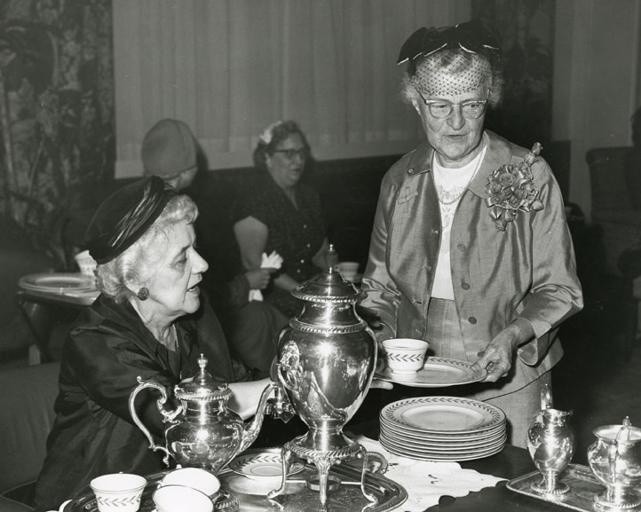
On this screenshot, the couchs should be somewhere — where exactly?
[0,359,67,512]
[44,149,400,272]
[586,147,640,357]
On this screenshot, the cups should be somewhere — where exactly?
[333,261,359,281]
[587,416,640,509]
[382,338,429,374]
[528,407,576,496]
[150,465,222,512]
[90,474,146,512]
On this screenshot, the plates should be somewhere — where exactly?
[378,394,507,463]
[229,477,304,496]
[228,453,304,480]
[375,356,487,388]
[18,273,94,294]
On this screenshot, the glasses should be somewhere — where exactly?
[416,85,488,119]
[275,147,307,159]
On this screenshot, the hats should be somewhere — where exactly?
[84,175,179,264]
[141,118,198,179]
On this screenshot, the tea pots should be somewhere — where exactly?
[131,354,276,469]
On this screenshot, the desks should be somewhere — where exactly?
[15,266,98,341]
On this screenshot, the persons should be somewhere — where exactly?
[75,118,291,384]
[30,174,394,512]
[226,122,365,316]
[356,21,584,453]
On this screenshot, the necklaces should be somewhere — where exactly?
[429,136,485,205]
